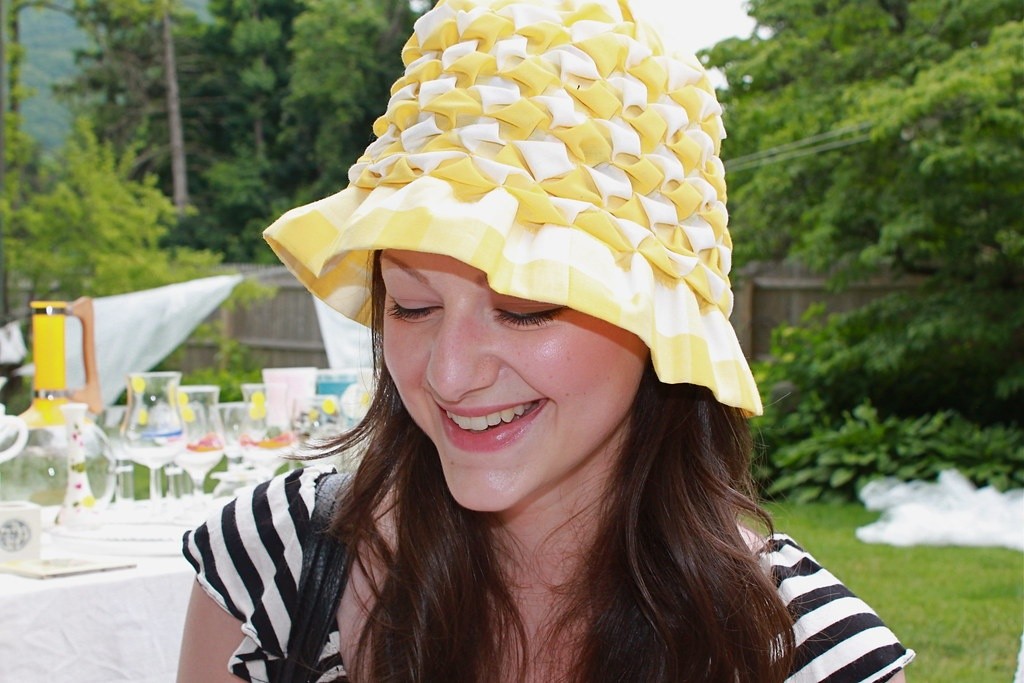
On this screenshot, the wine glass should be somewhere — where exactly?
[105,371,354,522]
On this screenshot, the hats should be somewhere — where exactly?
[263,1,762,419]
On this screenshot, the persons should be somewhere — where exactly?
[177,1,918,683]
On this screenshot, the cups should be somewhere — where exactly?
[262,367,317,427]
[55,403,116,531]
[0,504,41,565]
